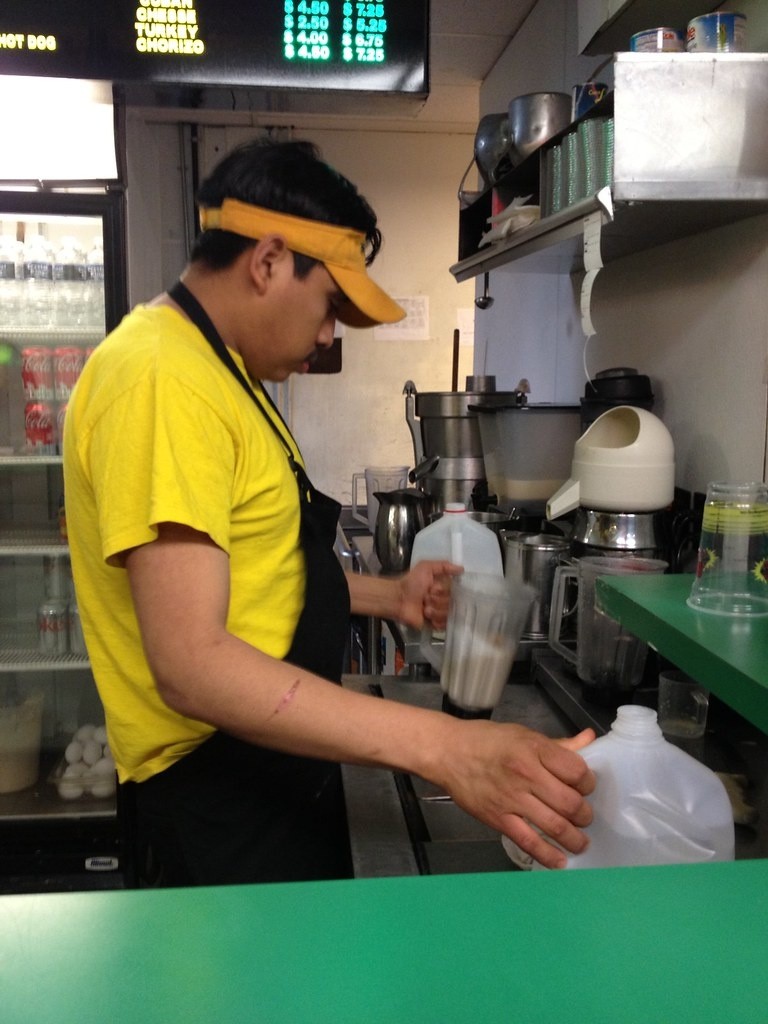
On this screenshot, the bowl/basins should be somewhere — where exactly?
[472,112,511,184]
[508,90,571,157]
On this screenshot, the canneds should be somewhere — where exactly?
[629,27,686,52]
[36,598,87,654]
[686,10,748,52]
[19,344,98,457]
[570,82,608,122]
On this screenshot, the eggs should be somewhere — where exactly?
[59,723,116,799]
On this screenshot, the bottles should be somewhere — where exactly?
[409,503,503,638]
[533,705,736,869]
[0,235,105,327]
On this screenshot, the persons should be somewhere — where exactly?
[62,140,595,889]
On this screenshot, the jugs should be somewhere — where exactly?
[499,526,579,640]
[351,465,410,534]
[372,488,431,575]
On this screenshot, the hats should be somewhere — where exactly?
[198,201,410,328]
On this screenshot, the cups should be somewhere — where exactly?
[418,570,537,713]
[1,686,46,792]
[658,667,710,739]
[685,481,768,618]
[546,555,669,691]
